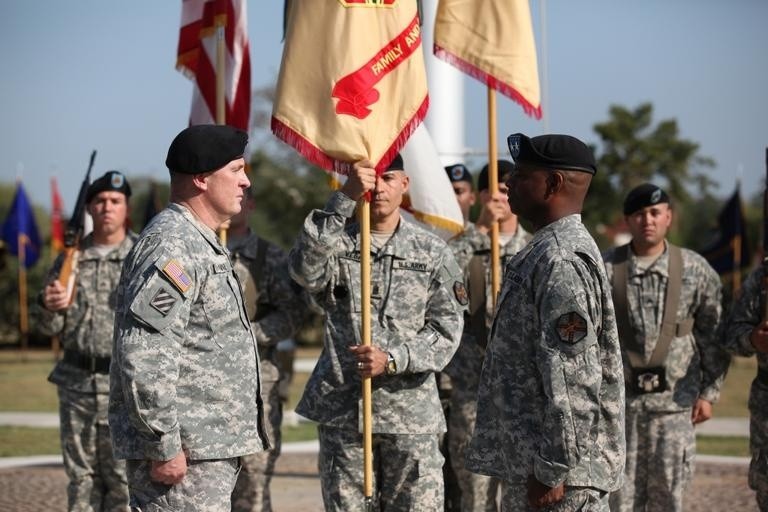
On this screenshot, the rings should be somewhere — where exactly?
[356,362,364,370]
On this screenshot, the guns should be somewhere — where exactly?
[758,145,767,323]
[59,150,97,304]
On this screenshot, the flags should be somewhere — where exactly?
[1,179,42,271]
[269,0,431,203]
[174,1,250,176]
[430,1,542,122]
[41,179,67,262]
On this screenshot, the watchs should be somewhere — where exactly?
[384,354,397,375]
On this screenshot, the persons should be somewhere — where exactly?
[108,124,268,511]
[463,132,625,512]
[227,186,316,510]
[598,184,733,512]
[288,149,470,512]
[435,162,475,230]
[717,250,767,512]
[445,157,527,512]
[28,170,140,512]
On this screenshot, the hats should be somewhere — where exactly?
[166,125,248,174]
[624,184,670,216]
[445,165,472,184]
[478,161,517,192]
[387,154,403,171]
[507,133,597,175]
[86,172,131,204]
